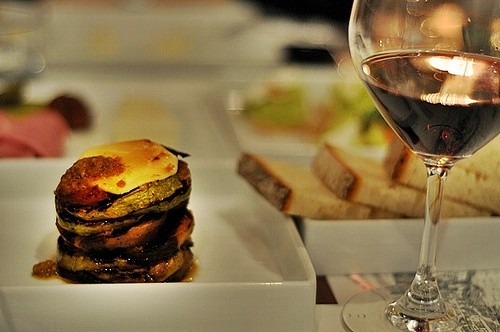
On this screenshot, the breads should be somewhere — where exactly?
[235,128,499,222]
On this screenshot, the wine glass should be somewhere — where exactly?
[339,2,500,331]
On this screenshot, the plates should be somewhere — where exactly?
[279,153,499,272]
[1,155,318,331]
